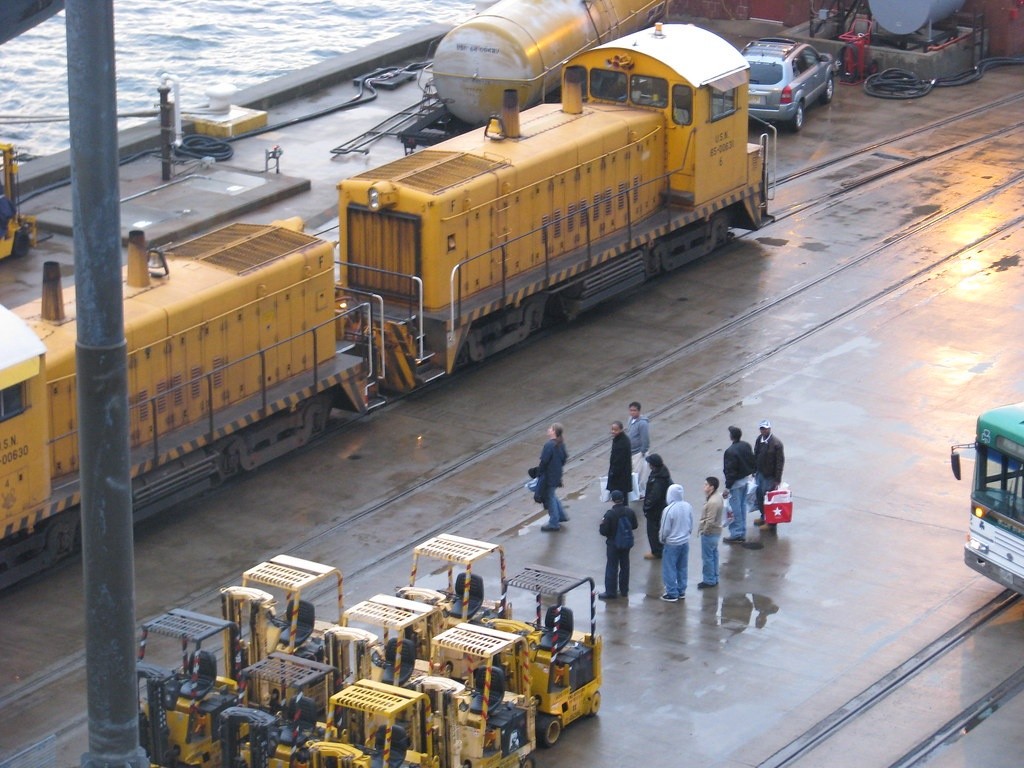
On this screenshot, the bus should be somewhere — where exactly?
[950,402,1024,601]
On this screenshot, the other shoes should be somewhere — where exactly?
[698,582,714,588]
[598,592,616,599]
[559,518,569,522]
[759,524,777,531]
[754,518,765,525]
[664,592,686,598]
[660,595,678,602]
[541,525,560,531]
[644,552,660,559]
[716,582,718,584]
[639,495,644,500]
[621,591,628,597]
[723,536,745,543]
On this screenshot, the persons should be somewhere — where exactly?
[598,490,639,600]
[754,420,785,532]
[659,484,694,602]
[526,422,571,532]
[624,402,652,503]
[696,477,724,589]
[606,420,633,507]
[723,425,757,545]
[642,454,673,561]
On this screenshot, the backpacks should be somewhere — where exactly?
[611,505,634,552]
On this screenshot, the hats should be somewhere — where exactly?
[728,426,742,440]
[608,491,624,500]
[645,454,663,467]
[759,419,771,429]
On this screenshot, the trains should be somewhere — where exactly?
[1,22,777,605]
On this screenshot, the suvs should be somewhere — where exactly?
[741,36,836,132]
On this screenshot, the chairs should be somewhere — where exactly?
[370,725,408,768]
[381,637,416,684]
[449,572,484,619]
[540,606,574,651]
[280,694,317,746]
[280,599,316,647]
[179,650,217,701]
[470,665,506,715]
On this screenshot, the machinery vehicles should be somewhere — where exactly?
[134,535,603,768]
[1,141,38,265]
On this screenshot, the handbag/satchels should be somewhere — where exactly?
[599,472,639,502]
[747,473,760,512]
[721,493,735,528]
[747,476,758,495]
[763,484,793,525]
